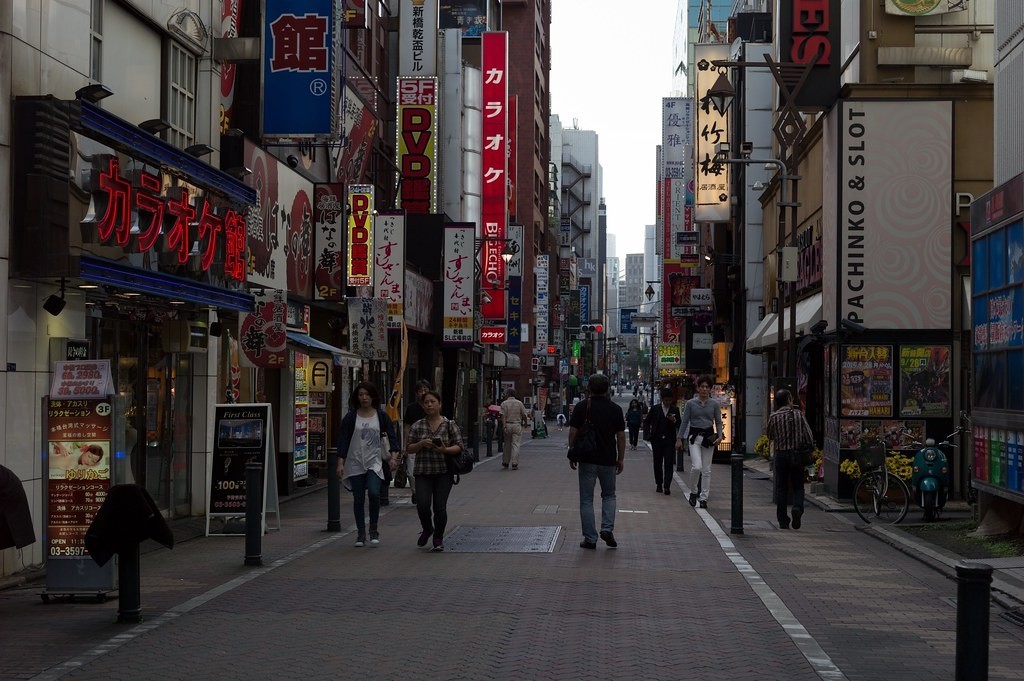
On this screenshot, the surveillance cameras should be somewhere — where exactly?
[483,297,491,304]
[740,142,753,155]
[483,291,493,299]
[714,142,730,154]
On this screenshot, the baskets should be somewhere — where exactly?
[853,444,885,468]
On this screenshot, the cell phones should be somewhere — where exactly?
[433,437,442,449]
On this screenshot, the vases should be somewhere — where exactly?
[858,489,914,505]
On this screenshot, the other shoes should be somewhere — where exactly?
[501,463,509,468]
[512,464,518,470]
[380,497,389,506]
[579,541,596,549]
[630,445,637,451]
[656,485,663,492]
[411,493,417,504]
[780,516,790,529]
[664,487,671,495]
[600,531,617,547]
[369,532,379,544]
[355,537,366,547]
[791,508,802,529]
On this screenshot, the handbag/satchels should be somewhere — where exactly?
[446,419,474,485]
[380,431,391,460]
[798,444,817,466]
[394,455,407,488]
[567,447,582,470]
[707,433,719,446]
[643,426,651,441]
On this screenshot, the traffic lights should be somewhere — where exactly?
[620,351,630,356]
[582,324,603,333]
[531,357,539,372]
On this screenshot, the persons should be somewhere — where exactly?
[638,391,645,402]
[568,373,626,549]
[515,391,524,426]
[643,388,682,495]
[675,374,723,509]
[126,418,137,483]
[407,391,465,551]
[766,388,814,529]
[403,379,431,504]
[625,399,644,450]
[49,443,104,470]
[634,383,638,395]
[336,382,402,546]
[499,388,527,470]
[531,403,539,419]
[557,414,566,432]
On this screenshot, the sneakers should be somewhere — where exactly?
[418,527,434,546]
[433,538,444,551]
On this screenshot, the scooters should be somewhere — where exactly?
[899,425,966,522]
[960,410,987,521]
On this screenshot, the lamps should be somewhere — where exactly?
[210,307,222,338]
[75,84,114,104]
[226,167,252,179]
[44,277,66,316]
[184,144,214,157]
[138,119,170,135]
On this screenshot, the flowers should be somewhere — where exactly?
[840,450,914,489]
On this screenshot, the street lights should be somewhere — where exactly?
[469,238,515,461]
[711,53,819,407]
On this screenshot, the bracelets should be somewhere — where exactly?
[392,457,398,461]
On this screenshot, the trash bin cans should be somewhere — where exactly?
[533,415,546,436]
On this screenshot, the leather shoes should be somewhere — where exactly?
[689,493,697,506]
[700,500,707,508]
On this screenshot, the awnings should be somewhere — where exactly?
[287,332,361,367]
[746,290,823,354]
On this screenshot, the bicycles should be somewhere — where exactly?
[853,432,910,524]
